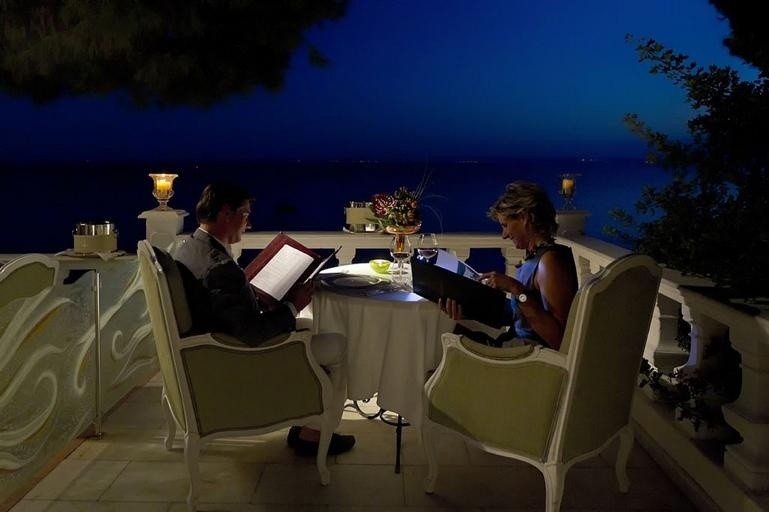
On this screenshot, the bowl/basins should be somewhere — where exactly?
[370,260,391,274]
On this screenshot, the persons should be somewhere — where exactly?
[161,176,358,458]
[436,178,582,357]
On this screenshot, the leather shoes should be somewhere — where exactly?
[287,425,356,457]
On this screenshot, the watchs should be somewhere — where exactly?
[513,291,532,306]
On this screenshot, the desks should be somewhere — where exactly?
[56,256,140,439]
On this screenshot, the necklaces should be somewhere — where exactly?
[513,241,550,269]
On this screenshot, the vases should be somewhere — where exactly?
[385,223,422,264]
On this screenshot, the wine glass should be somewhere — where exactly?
[392,234,413,282]
[416,233,438,263]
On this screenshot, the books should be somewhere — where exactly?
[409,248,508,334]
[243,230,344,310]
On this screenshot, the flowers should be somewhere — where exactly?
[364,170,446,232]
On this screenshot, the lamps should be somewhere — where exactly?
[147,173,179,211]
[558,176,577,212]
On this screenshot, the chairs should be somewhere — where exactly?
[421,253,663,510]
[139,240,335,511]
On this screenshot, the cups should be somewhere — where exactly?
[77,216,115,258]
[349,201,375,233]
[392,259,412,291]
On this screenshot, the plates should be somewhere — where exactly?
[322,273,391,292]
